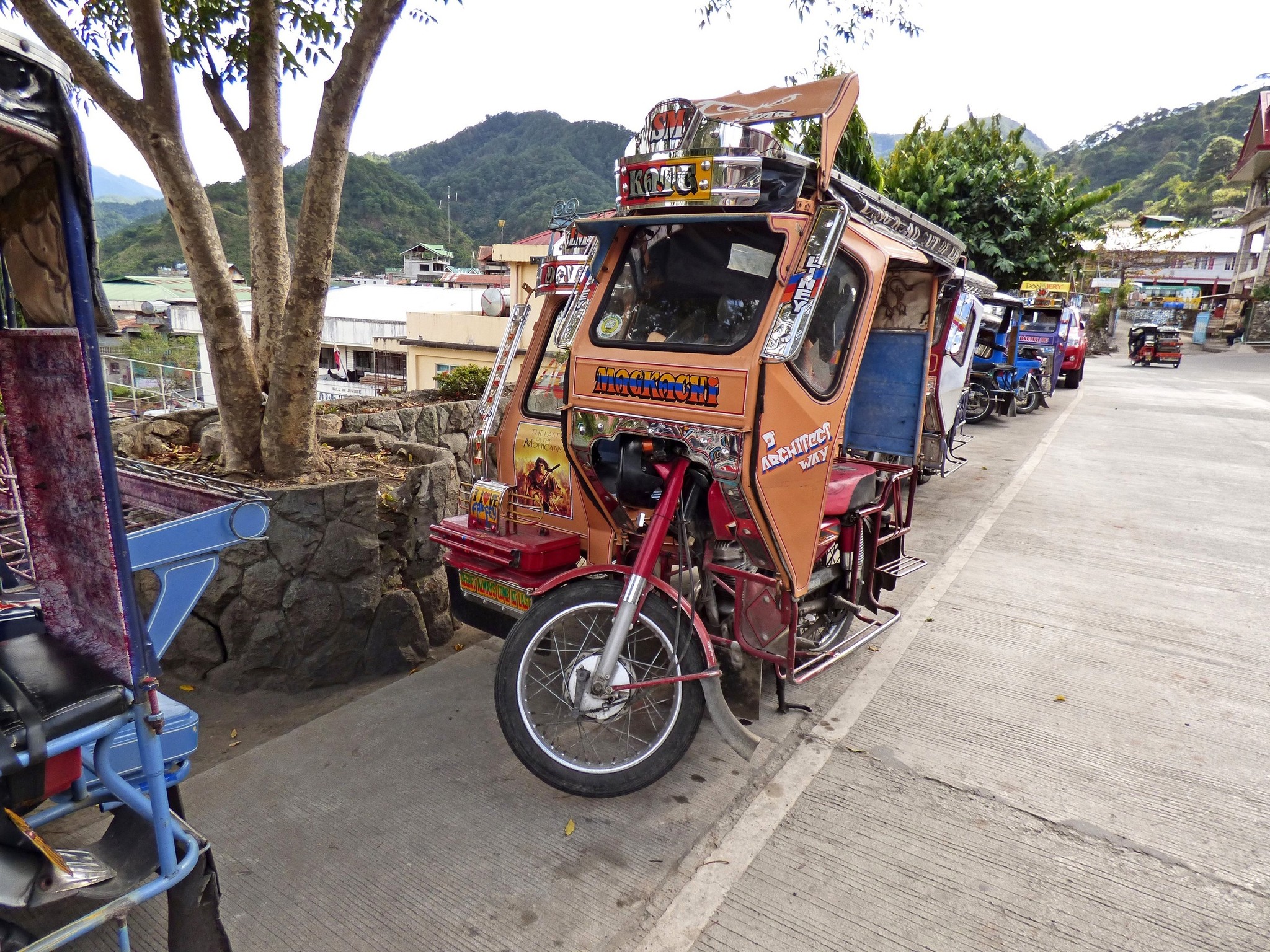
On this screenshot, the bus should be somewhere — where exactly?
[1122,282,1203,310]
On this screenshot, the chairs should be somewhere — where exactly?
[1233,333,1244,343]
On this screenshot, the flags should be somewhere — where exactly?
[334,343,340,371]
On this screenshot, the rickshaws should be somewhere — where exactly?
[1127,322,1184,369]
[426,72,965,799]
[838,266,1073,512]
[0,23,277,952]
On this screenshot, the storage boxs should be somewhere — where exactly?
[1163,302,1184,309]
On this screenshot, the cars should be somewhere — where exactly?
[1059,305,1088,389]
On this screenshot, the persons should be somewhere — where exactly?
[1127,288,1147,306]
[1225,322,1244,346]
[1213,304,1224,318]
